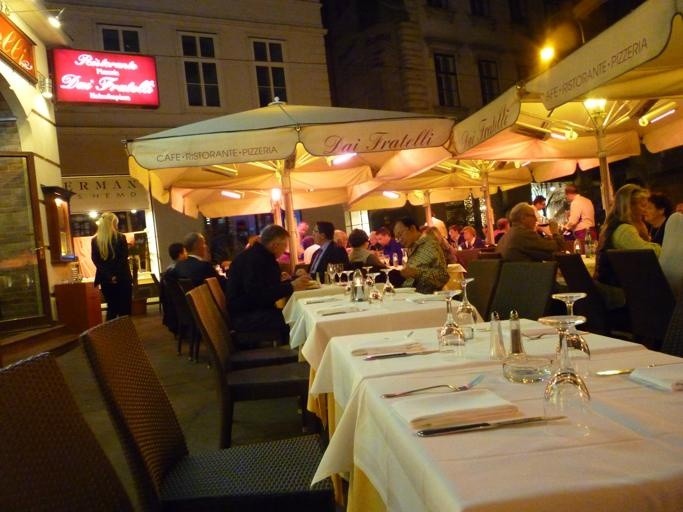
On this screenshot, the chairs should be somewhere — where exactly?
[185,284,322,448]
[599,249,675,358]
[557,251,638,342]
[151,264,217,364]
[661,300,682,357]
[1,351,136,512]
[301,243,558,321]
[81,316,336,511]
[206,277,292,348]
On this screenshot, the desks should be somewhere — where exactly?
[312,318,682,512]
[284,285,475,429]
[556,252,598,288]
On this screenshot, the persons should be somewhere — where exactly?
[89,212,133,320]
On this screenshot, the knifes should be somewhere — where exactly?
[362,347,434,363]
[595,362,678,377]
[417,412,564,439]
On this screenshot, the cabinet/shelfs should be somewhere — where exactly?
[54,282,103,336]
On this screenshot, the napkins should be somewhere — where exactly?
[351,333,423,356]
[304,294,346,304]
[404,293,444,303]
[316,304,366,316]
[630,363,681,393]
[515,319,556,337]
[391,390,520,428]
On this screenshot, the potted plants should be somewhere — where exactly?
[129,240,147,316]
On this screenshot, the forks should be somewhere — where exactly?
[523,330,590,341]
[381,373,486,400]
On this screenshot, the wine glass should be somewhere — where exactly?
[376,246,408,267]
[549,291,590,366]
[452,277,476,338]
[537,315,592,436]
[325,260,396,304]
[434,287,467,359]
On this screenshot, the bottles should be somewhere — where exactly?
[488,310,505,362]
[570,220,598,257]
[508,306,526,358]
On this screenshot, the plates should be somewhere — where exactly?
[393,288,415,292]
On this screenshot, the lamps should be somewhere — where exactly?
[21,64,53,100]
[3,4,66,29]
